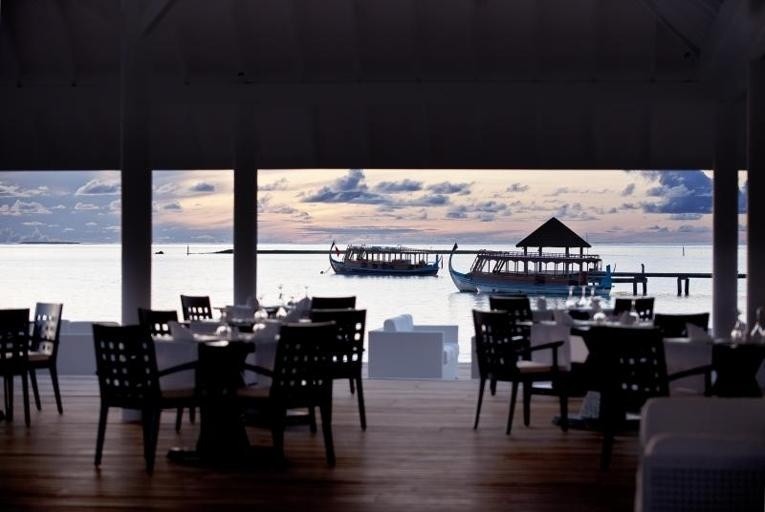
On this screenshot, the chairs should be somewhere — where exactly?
[469,281,765,398]
[0,293,66,426]
[93,324,200,471]
[471,308,568,436]
[143,295,368,436]
[226,319,335,467]
[589,323,719,489]
[26,312,117,377]
[366,308,462,384]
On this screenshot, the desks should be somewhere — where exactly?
[635,399,765,512]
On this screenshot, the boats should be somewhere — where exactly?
[447,241,613,298]
[327,238,441,276]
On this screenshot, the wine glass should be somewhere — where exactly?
[212,283,310,340]
[562,278,764,347]
